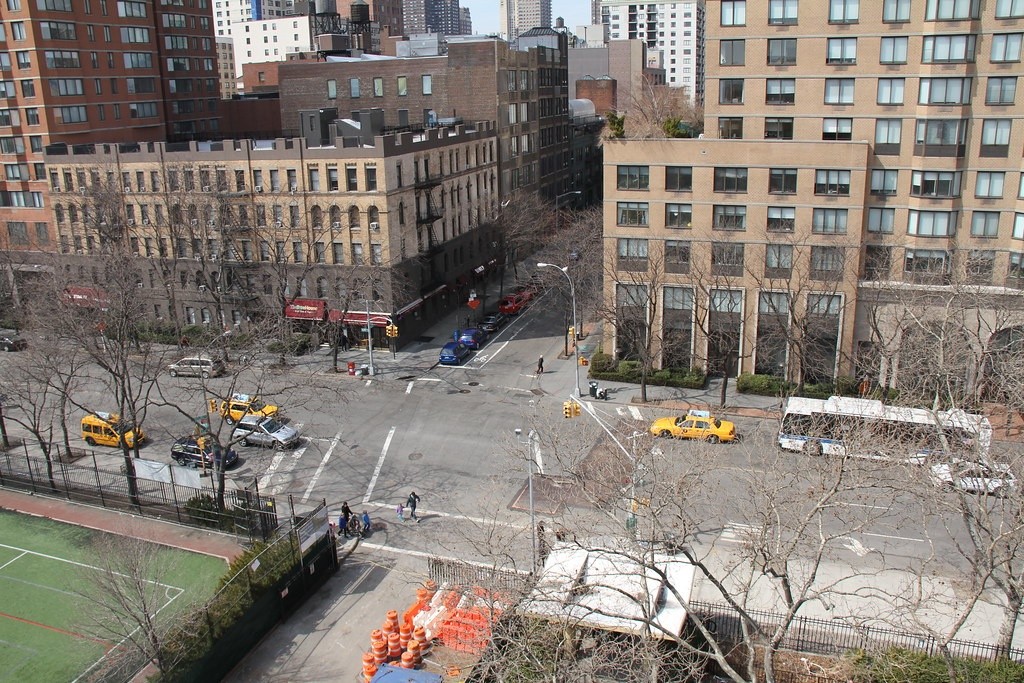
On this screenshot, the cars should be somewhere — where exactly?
[170,434,239,470]
[478,249,580,330]
[930,457,1023,498]
[438,339,471,365]
[458,326,490,350]
[649,410,737,445]
[218,392,278,425]
[232,414,300,452]
[0,330,28,352]
[80,410,146,448]
[167,354,227,379]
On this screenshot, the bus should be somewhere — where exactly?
[776,393,993,467]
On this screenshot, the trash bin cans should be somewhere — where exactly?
[348,362,355,375]
[588,381,599,396]
[360,364,368,375]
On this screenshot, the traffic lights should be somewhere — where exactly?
[210,399,218,413]
[385,323,398,337]
[568,327,574,336]
[563,401,581,418]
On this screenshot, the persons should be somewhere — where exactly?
[343,334,348,351]
[181,335,190,349]
[406,492,420,523]
[396,504,406,523]
[536,355,544,374]
[338,501,370,539]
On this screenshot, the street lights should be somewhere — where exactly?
[514,427,538,586]
[537,262,579,388]
[554,189,582,251]
[197,330,232,482]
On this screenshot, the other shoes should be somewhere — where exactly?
[337,532,340,536]
[345,536,349,538]
[363,530,367,532]
[367,529,370,532]
[347,531,351,535]
